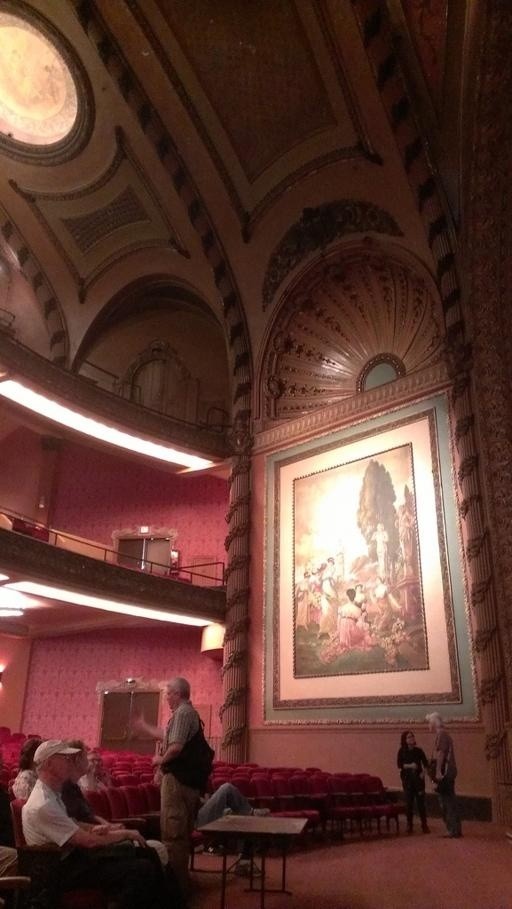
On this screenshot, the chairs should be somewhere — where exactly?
[1,724,410,881]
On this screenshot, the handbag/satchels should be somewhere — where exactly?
[427,760,445,784]
[167,731,215,792]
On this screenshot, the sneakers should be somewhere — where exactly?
[203,847,232,856]
[249,807,270,817]
[234,862,261,878]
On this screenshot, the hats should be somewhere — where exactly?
[33,739,81,765]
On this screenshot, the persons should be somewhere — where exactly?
[11,737,47,801]
[423,710,467,839]
[77,748,112,799]
[396,728,432,835]
[1,843,20,907]
[188,781,273,878]
[148,674,216,840]
[60,738,128,835]
[295,503,420,665]
[22,738,148,849]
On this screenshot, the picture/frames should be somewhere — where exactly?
[266,400,468,712]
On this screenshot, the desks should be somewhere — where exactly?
[190,809,314,908]
